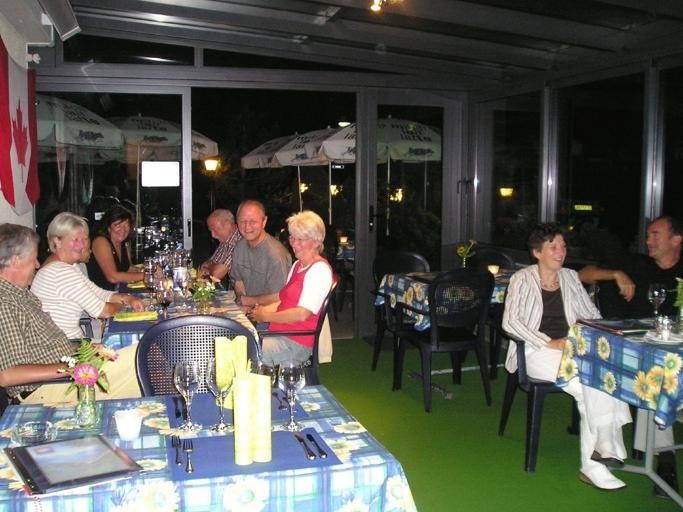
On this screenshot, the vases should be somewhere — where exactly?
[75,390,98,427]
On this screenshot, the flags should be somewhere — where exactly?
[0,36,42,215]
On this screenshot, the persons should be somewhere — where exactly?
[499,222,633,491]
[223,198,292,331]
[199,208,242,285]
[256,209,335,369]
[73,238,93,280]
[28,210,143,349]
[85,207,158,292]
[0,222,177,407]
[578,213,683,500]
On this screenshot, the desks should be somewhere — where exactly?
[0,385,417,512]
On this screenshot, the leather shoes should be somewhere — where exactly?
[655,451,679,499]
[577,439,627,490]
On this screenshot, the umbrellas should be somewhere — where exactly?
[241,132,308,217]
[316,114,443,239]
[270,125,346,227]
[32,95,124,216]
[74,115,219,228]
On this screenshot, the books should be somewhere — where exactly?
[2,434,142,497]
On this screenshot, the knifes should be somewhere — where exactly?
[306,434,327,459]
[293,431,315,461]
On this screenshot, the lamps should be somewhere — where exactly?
[369,0,386,12]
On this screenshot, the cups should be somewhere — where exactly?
[655,316,672,341]
[113,409,141,442]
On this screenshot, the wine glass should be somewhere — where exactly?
[205,359,234,435]
[246,358,276,431]
[173,361,202,433]
[278,359,306,433]
[646,283,665,328]
[142,214,193,321]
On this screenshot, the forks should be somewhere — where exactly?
[171,435,182,466]
[182,439,193,474]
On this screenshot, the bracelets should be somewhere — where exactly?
[121,293,127,306]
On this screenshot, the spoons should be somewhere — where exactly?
[272,392,287,411]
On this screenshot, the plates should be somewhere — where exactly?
[645,332,682,346]
